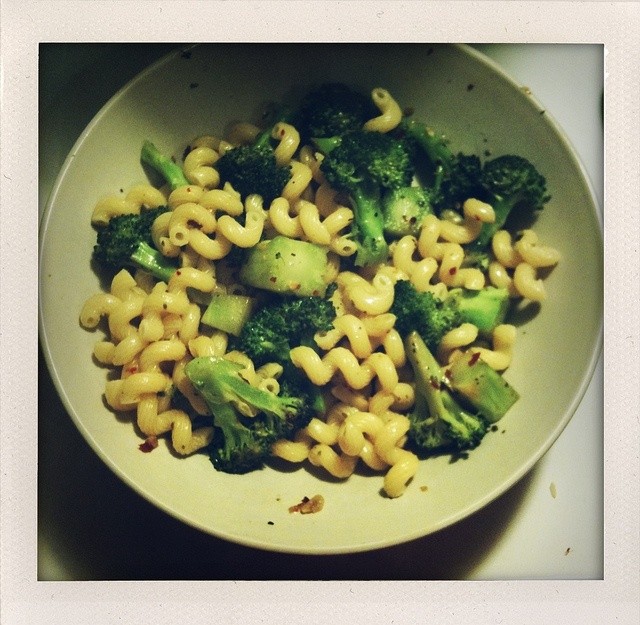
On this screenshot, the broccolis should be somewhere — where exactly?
[214,144,293,207]
[231,294,337,371]
[390,279,465,345]
[444,152,550,257]
[320,128,416,264]
[92,203,177,279]
[405,329,489,456]
[293,81,382,135]
[141,139,211,227]
[183,354,314,474]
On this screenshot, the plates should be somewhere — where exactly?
[40,43,603,555]
